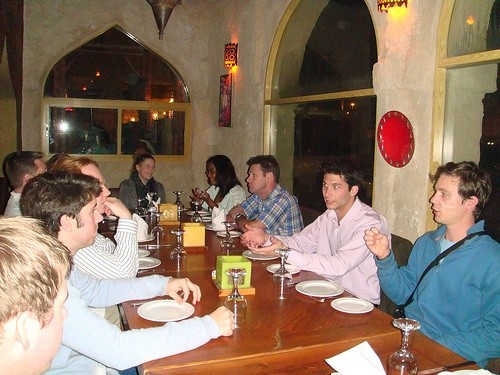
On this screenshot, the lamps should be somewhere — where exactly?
[377,0,408,14]
[224,42,238,69]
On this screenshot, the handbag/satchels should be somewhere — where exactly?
[393,305,406,320]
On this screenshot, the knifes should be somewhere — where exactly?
[418,360,478,375]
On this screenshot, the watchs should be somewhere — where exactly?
[234,212,244,222]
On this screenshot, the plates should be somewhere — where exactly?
[295,278,345,298]
[187,210,235,231]
[137,249,150,258]
[137,299,195,323]
[137,234,155,242]
[330,297,374,314]
[266,264,300,276]
[101,215,118,230]
[217,230,243,238]
[243,246,280,260]
[137,257,162,270]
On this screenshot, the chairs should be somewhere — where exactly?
[379,233,413,315]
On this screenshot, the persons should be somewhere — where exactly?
[0,217,71,375]
[225,154,305,238]
[362,161,500,369]
[16,170,236,375]
[118,153,166,222]
[0,150,138,318]
[240,158,392,310]
[189,155,247,216]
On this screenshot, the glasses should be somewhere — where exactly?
[205,170,216,174]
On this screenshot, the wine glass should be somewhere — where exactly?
[172,190,185,215]
[223,268,249,323]
[145,192,158,214]
[151,211,165,240]
[388,318,421,375]
[190,199,204,224]
[168,230,187,265]
[219,221,236,256]
[272,249,293,299]
[134,198,146,221]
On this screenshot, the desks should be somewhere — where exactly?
[100,207,494,374]
